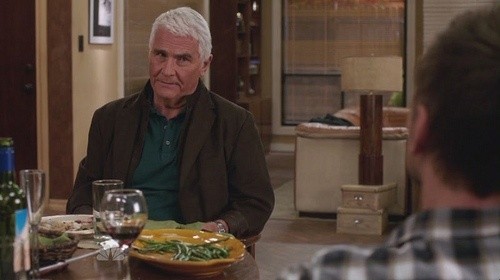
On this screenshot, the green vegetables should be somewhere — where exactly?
[37,233,71,245]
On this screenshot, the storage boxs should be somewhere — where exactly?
[340,182,398,210]
[337,205,392,236]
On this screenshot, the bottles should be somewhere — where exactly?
[0,137,31,280]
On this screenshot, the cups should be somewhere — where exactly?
[92,179,124,244]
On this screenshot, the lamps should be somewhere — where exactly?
[341,52,403,186]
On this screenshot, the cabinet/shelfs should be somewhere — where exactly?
[209,0,264,153]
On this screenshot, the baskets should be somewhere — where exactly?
[39,228,80,272]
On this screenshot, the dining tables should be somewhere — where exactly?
[33,207,260,280]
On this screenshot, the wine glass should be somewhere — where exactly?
[17,169,50,280]
[100,189,148,280]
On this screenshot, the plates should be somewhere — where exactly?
[39,213,95,238]
[127,217,246,276]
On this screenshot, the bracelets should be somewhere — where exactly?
[214,221,226,234]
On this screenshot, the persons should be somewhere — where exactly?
[275,7,500,280]
[66,6,275,239]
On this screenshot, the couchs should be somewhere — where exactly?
[293,101,411,219]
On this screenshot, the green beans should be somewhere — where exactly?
[133,238,230,261]
